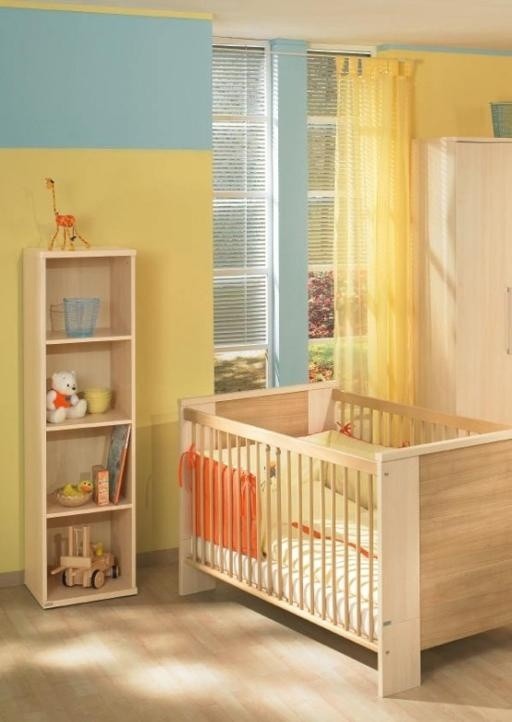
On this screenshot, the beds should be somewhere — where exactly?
[178,380,512,698]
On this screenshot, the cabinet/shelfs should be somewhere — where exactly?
[23,244,138,611]
[409,136,511,438]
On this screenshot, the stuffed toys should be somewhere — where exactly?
[46,371,88,423]
[64,479,94,496]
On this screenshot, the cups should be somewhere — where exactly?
[63,297,99,338]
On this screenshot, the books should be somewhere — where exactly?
[92,465,109,505]
[107,424,131,505]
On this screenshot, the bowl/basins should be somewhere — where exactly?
[54,485,93,506]
[79,387,113,414]
